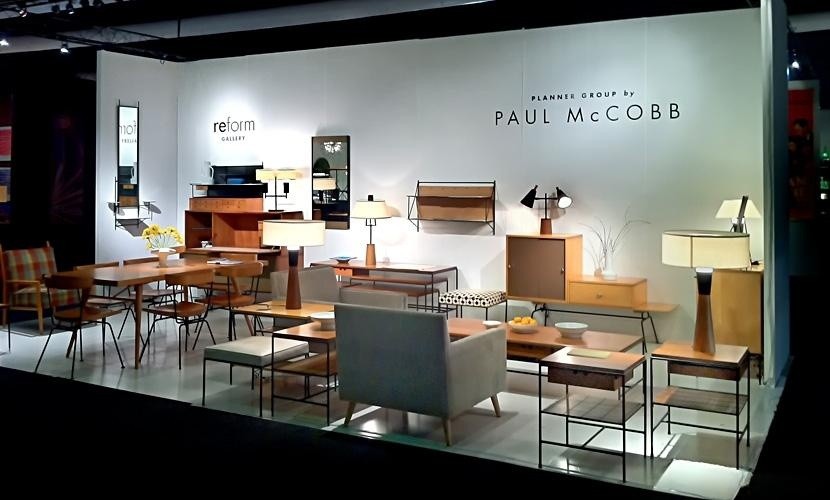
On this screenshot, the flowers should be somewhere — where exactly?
[142,225,185,247]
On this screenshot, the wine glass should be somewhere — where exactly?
[310,312,335,331]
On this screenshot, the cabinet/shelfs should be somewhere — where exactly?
[699,260,765,385]
[310,255,461,318]
[184,209,305,278]
[501,232,681,346]
[405,179,498,236]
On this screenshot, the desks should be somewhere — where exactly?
[650,341,755,472]
[188,180,264,210]
[313,198,347,220]
[534,348,652,484]
[270,321,339,428]
[119,182,138,207]
[232,298,648,424]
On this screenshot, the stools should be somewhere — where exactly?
[438,288,508,321]
[200,337,309,419]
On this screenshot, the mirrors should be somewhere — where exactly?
[311,134,352,231]
[116,100,141,227]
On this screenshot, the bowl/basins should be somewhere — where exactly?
[509,320,534,333]
[555,322,588,339]
[482,320,501,328]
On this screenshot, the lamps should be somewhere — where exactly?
[715,197,762,233]
[16,0,106,20]
[519,183,573,234]
[0,33,71,56]
[262,218,327,309]
[351,194,392,267]
[661,228,753,354]
[255,167,301,212]
[313,177,337,201]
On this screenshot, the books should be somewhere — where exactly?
[206,257,229,264]
[201,240,212,248]
[566,348,612,359]
[219,259,244,265]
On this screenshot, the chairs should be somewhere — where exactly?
[119,166,134,183]
[332,304,509,448]
[210,165,263,184]
[1,246,267,377]
[314,209,347,228]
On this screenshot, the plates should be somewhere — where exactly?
[331,256,357,263]
